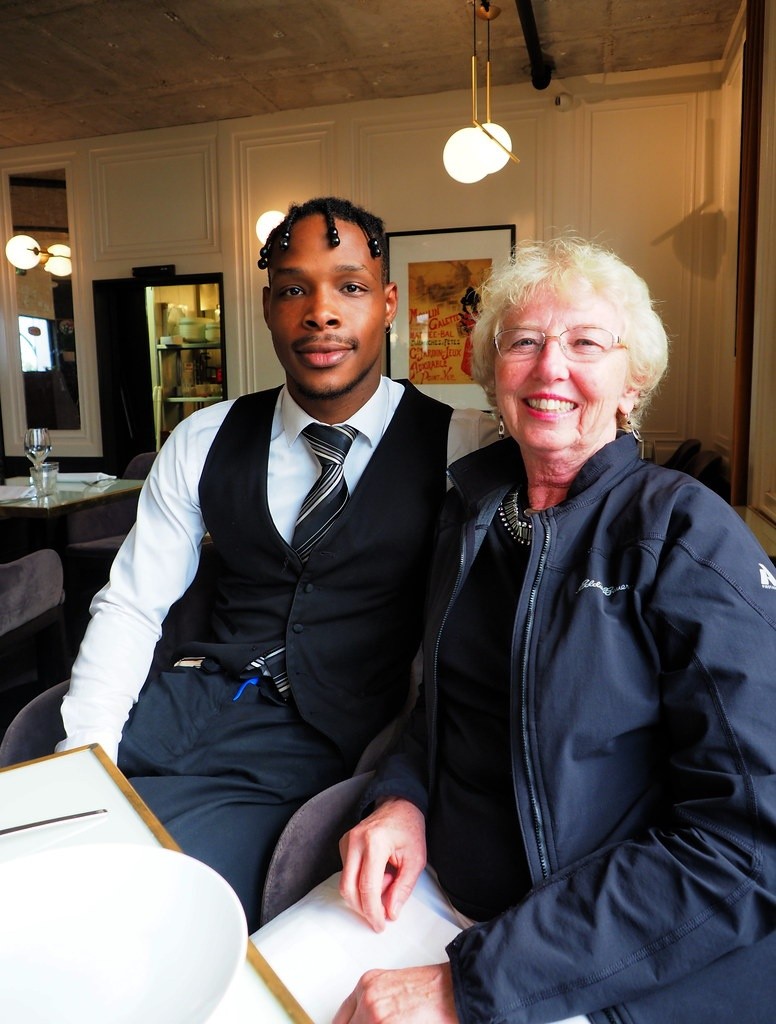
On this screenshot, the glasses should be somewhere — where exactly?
[492,326,630,361]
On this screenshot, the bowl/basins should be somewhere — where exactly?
[177,317,220,343]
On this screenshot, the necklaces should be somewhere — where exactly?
[498,488,533,549]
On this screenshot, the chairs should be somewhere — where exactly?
[0,452,432,924]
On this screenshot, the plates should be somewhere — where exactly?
[1,844,249,1024]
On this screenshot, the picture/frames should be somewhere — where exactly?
[384,223,517,413]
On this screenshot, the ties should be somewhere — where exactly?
[246,423,359,702]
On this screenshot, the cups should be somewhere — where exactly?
[181,384,222,398]
[29,461,59,494]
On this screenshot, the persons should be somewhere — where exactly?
[30,197,511,935]
[456,285,483,383]
[340,220,776,1024]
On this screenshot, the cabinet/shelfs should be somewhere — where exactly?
[157,340,223,433]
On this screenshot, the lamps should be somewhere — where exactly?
[256,209,286,246]
[6,235,72,277]
[443,0,521,184]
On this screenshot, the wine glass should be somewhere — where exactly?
[23,428,53,501]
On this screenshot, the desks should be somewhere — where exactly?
[0,477,144,554]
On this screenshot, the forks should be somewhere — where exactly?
[81,478,119,487]
